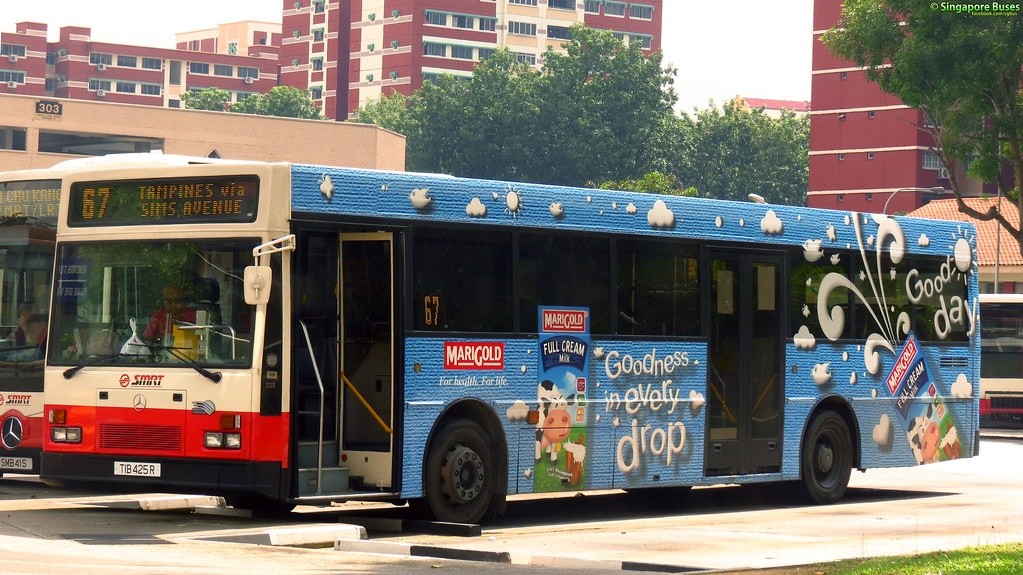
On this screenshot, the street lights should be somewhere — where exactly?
[882,187,945,215]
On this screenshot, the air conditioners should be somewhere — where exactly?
[97,89,106,97]
[245,77,253,84]
[291,2,301,66]
[8,54,18,63]
[7,81,18,89]
[97,63,107,72]
[366,9,399,80]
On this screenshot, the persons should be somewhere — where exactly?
[8,301,47,346]
[141,283,196,339]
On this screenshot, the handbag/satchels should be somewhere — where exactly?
[119,317,154,361]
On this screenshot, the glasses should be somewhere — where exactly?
[162,296,185,305]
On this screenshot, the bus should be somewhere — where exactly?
[0,152,1023,524]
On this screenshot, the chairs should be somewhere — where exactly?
[190,278,222,330]
